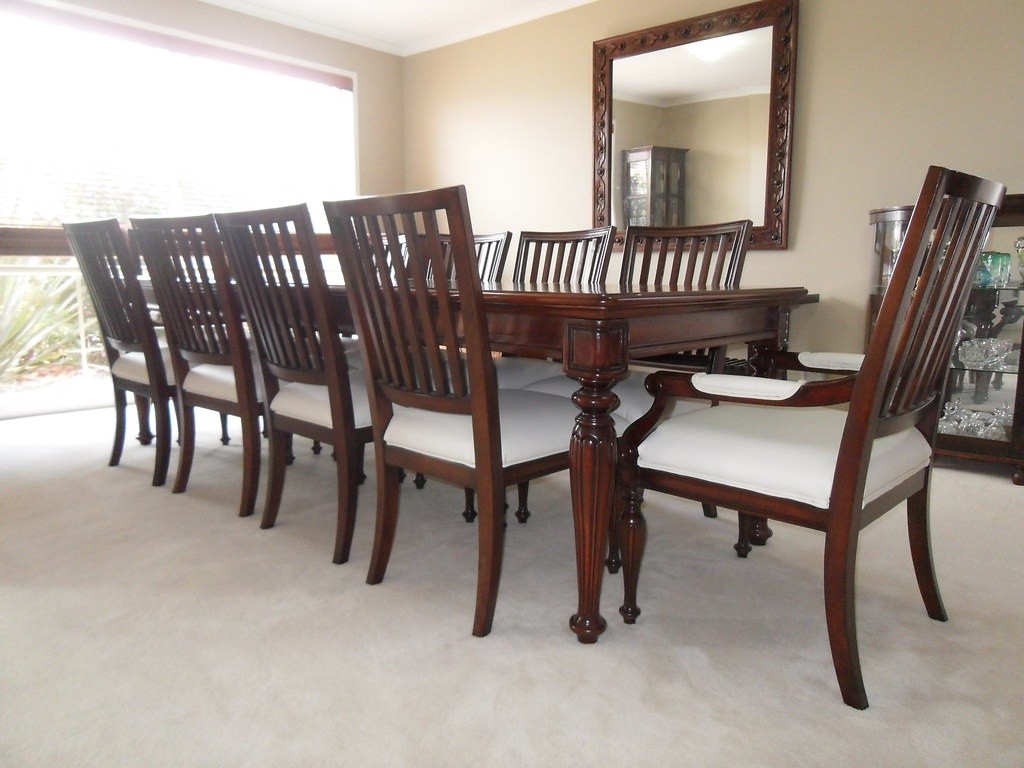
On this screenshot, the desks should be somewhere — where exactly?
[111,271,819,645]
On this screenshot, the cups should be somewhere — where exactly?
[972,252,1012,287]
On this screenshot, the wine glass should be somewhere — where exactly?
[936,399,1011,441]
[957,337,1014,370]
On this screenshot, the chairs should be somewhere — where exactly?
[60,163,1008,708]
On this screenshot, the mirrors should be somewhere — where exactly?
[591,0,799,252]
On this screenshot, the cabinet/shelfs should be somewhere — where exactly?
[620,145,690,227]
[864,189,1024,487]
